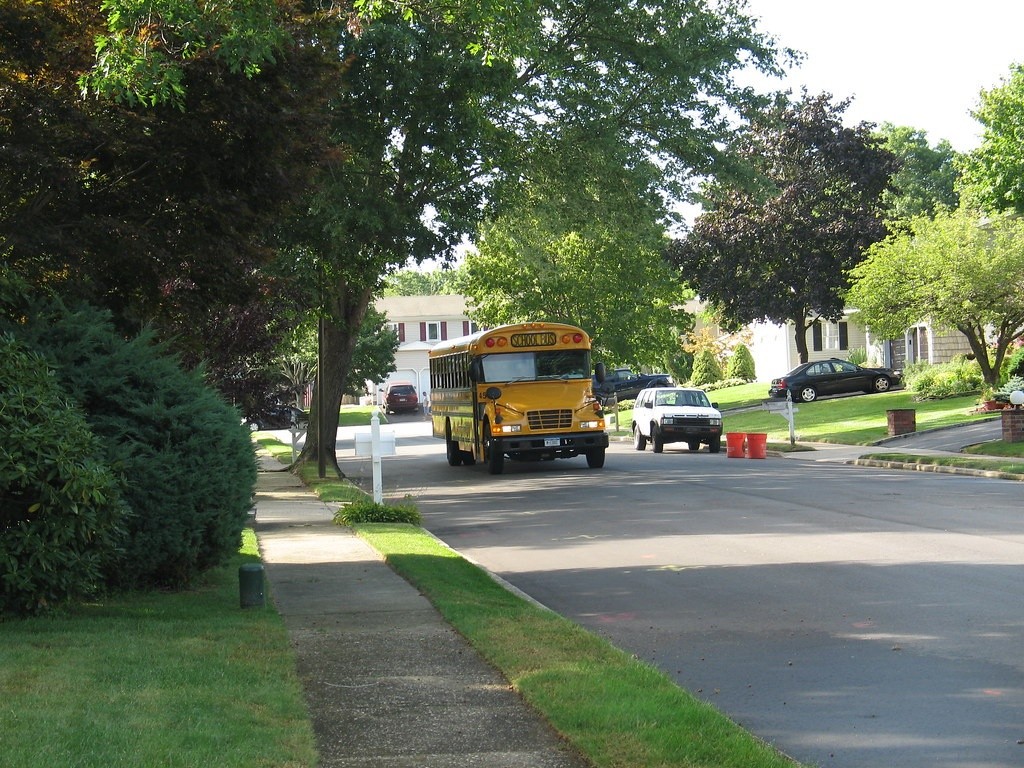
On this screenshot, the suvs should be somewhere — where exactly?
[379,382,420,416]
[590,367,676,406]
[631,386,723,453]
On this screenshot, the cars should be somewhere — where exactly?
[236,404,310,432]
[768,359,902,403]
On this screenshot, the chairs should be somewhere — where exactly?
[821,367,829,375]
[675,393,691,405]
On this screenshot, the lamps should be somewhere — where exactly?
[1010,391,1024,409]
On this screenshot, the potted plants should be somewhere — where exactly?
[982,389,996,411]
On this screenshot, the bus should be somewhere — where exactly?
[428,321,610,472]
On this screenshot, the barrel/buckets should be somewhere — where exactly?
[747,433,767,459]
[725,432,747,458]
